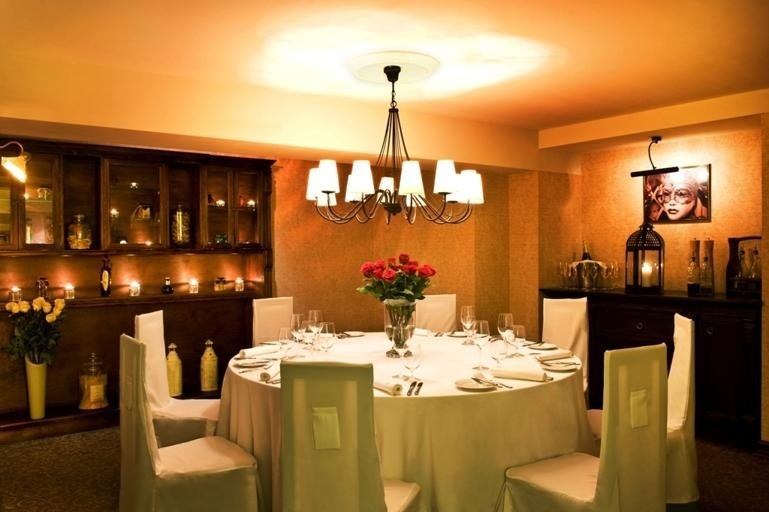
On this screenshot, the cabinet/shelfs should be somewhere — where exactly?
[0,131,276,447]
[540,287,760,448]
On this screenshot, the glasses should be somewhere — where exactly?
[661,189,695,204]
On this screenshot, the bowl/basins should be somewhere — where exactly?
[558,260,616,289]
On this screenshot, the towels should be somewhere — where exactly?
[492,365,546,381]
[373,379,402,395]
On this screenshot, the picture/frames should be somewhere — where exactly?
[643,164,711,224]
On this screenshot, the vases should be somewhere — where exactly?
[383,300,414,359]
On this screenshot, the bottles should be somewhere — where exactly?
[76,351,111,412]
[170,203,193,247]
[66,214,94,249]
[686,255,700,296]
[199,339,220,392]
[582,239,592,261]
[166,341,184,398]
[747,247,761,294]
[160,277,174,294]
[213,276,227,292]
[697,257,715,296]
[100,254,114,296]
[734,247,749,295]
[37,276,48,301]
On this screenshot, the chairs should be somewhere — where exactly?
[496,343,669,512]
[408,294,456,333]
[119,333,259,512]
[588,313,700,505]
[281,361,420,512]
[252,296,293,348]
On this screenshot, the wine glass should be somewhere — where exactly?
[279,308,525,398]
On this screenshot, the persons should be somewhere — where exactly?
[659,170,707,220]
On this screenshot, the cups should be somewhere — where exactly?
[189,283,199,294]
[235,281,244,292]
[64,288,75,300]
[9,291,23,304]
[128,286,141,297]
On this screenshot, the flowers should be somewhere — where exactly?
[356,254,436,346]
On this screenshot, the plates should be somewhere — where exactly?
[231,339,280,385]
[514,336,579,373]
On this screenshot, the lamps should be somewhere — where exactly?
[306,49,486,224]
[630,136,678,177]
[624,221,664,294]
